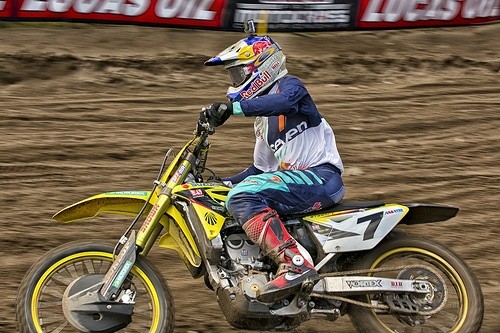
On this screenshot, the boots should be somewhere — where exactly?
[242,207,320,302]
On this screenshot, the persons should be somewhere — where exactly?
[199,35,344,302]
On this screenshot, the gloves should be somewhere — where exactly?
[205,102,233,128]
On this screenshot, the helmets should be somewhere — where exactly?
[204,34,289,100]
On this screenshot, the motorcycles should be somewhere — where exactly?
[14,104,484,333]
